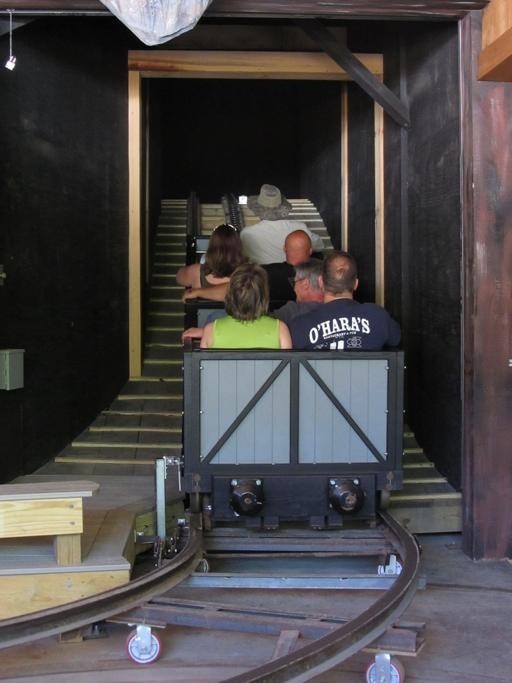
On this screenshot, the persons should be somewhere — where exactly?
[200,261,293,350]
[290,251,402,349]
[175,223,250,287]
[179,258,325,341]
[182,231,323,305]
[240,183,324,265]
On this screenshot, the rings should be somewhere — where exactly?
[189,288,192,292]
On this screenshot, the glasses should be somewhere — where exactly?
[288,276,305,288]
[213,224,239,231]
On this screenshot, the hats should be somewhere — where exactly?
[247,184,292,221]
